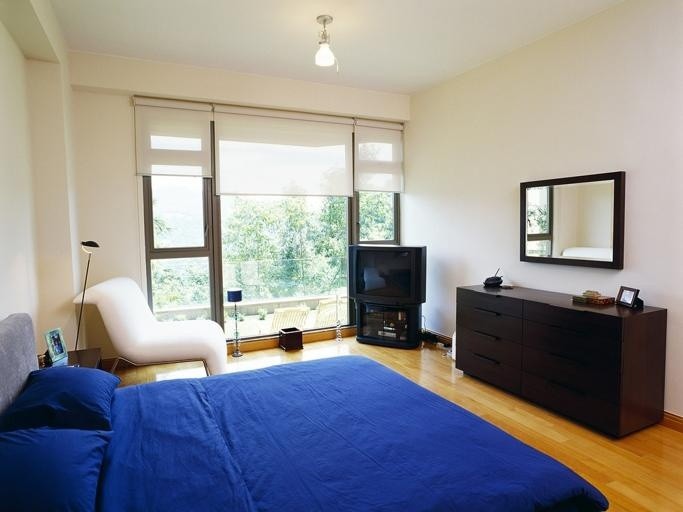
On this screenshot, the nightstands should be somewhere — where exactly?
[40,346,104,371]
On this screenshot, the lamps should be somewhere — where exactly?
[75,241,100,352]
[314,14,335,67]
[227,288,243,357]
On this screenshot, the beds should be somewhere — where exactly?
[0,311,608,512]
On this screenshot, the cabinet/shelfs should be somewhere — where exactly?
[455,284,668,440]
[354,296,422,349]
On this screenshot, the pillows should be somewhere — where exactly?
[0,367,122,430]
[1,429,113,511]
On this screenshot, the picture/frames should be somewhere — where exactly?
[616,285,640,308]
[43,328,68,363]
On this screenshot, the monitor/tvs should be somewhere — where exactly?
[348,242,427,305]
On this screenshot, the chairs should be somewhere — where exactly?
[73,276,228,376]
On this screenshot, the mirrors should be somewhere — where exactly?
[518,171,625,270]
